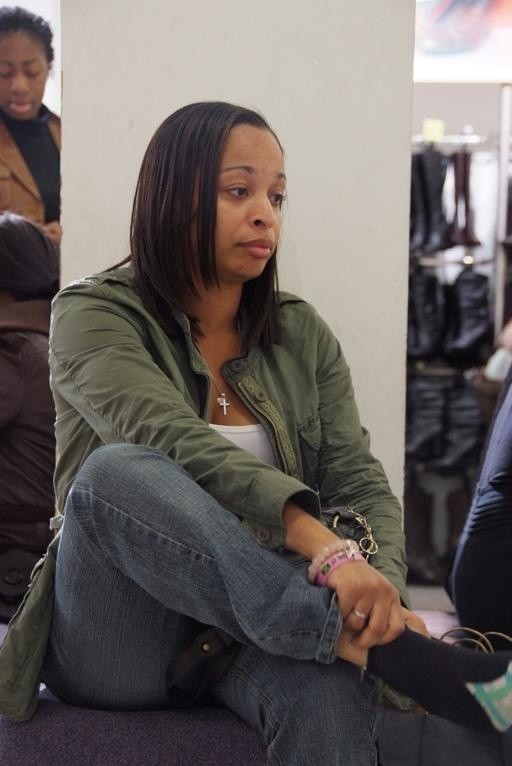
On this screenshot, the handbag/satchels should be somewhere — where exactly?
[308,539,366,586]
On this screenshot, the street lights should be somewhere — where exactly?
[164,505,378,706]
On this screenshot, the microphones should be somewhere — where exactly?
[0,606,465,766]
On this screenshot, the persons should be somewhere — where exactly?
[42,102,512,766]
[0,7,61,624]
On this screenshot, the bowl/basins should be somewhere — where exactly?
[194,341,244,417]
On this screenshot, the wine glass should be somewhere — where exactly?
[354,609,367,620]
